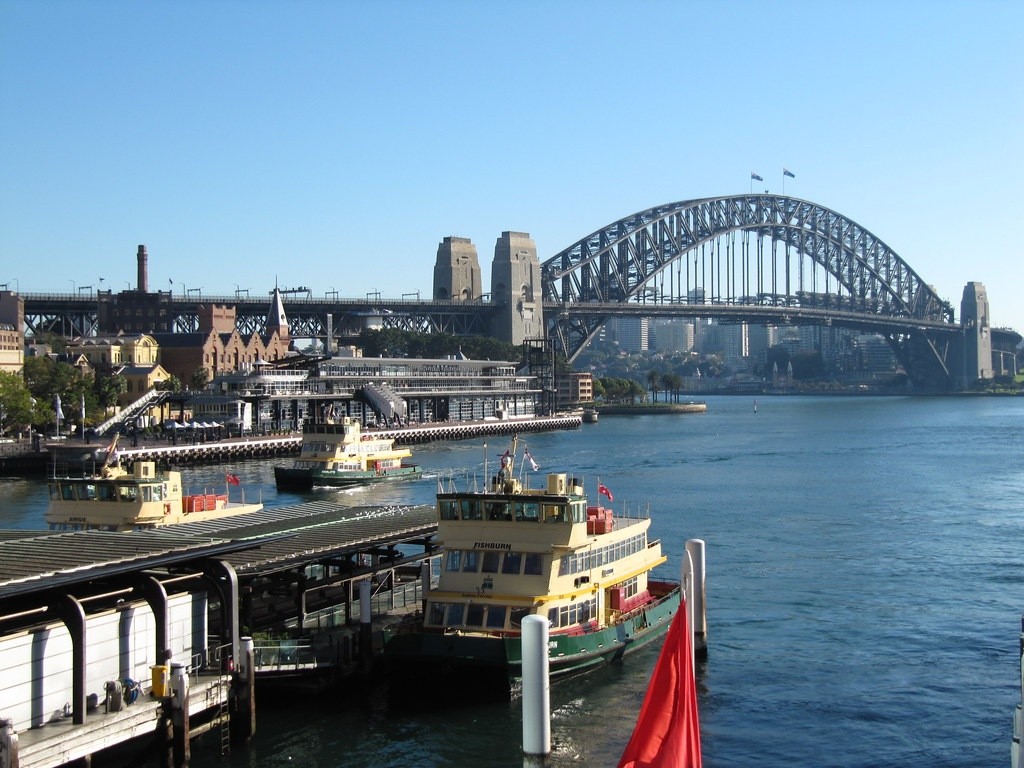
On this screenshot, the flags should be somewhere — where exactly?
[783,168,795,178]
[617,601,701,768]
[226,472,240,485]
[599,481,613,501]
[524,448,540,471]
[82,396,85,418]
[57,396,65,419]
[751,172,763,181]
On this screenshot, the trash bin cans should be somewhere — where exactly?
[149,666,168,696]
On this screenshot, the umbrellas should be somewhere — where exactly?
[166,421,224,437]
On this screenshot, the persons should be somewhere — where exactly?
[153,490,160,501]
[85,430,90,444]
[505,510,512,520]
[240,624,297,665]
[486,509,497,520]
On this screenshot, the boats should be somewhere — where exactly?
[421,433,683,703]
[273,403,423,493]
[44,431,264,531]
